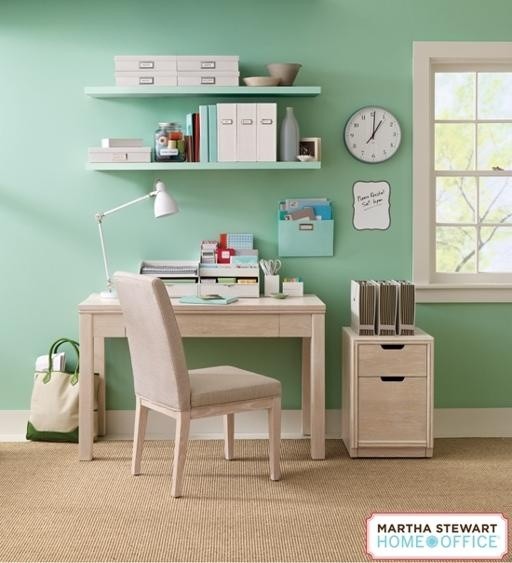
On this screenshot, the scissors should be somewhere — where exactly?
[269,259,281,275]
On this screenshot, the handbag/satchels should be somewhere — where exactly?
[25,371,101,443]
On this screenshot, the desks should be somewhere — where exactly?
[77,293,327,461]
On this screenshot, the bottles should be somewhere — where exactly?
[280,106,301,161]
[154,122,186,161]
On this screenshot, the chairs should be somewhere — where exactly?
[112,271,282,498]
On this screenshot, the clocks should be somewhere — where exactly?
[343,107,403,165]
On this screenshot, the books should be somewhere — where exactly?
[35,352,65,372]
[177,293,239,305]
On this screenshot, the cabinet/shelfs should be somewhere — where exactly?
[81,87,323,172]
[341,326,434,458]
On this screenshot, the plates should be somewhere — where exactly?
[270,292,289,299]
[242,76,280,86]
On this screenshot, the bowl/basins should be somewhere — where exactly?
[296,155,311,161]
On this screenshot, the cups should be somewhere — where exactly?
[263,274,281,297]
[267,63,302,85]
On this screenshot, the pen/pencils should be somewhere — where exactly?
[283,276,303,282]
[260,259,271,275]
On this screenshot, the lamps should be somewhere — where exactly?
[95,179,179,299]
